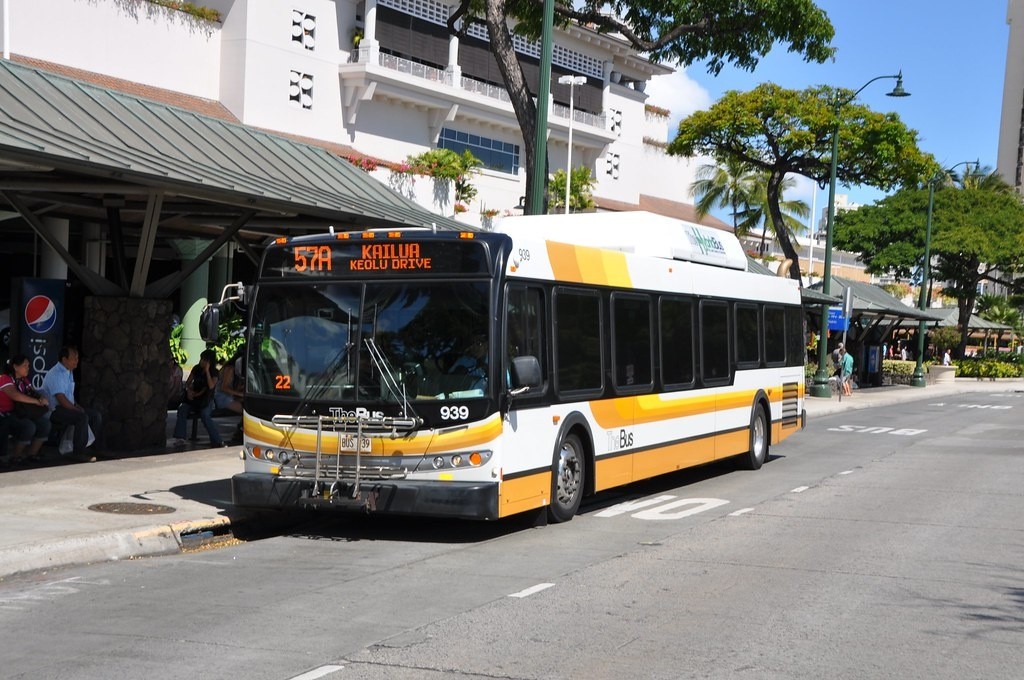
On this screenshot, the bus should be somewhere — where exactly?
[964,330,1021,362]
[198,210,810,529]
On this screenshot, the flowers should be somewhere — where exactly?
[341,150,512,221]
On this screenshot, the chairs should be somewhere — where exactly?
[398,372,488,397]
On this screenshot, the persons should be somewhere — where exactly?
[214,343,247,415]
[889,345,893,360]
[901,346,907,361]
[39,346,97,462]
[831,343,853,397]
[0,353,51,469]
[944,349,952,366]
[353,315,392,364]
[167,353,183,409]
[169,350,227,447]
[463,328,488,377]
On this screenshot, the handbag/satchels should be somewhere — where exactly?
[11,379,49,420]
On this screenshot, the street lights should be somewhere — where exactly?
[908,158,986,387]
[557,74,587,216]
[806,67,912,399]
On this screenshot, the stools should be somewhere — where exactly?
[186,411,202,442]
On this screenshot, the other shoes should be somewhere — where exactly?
[213,441,227,448]
[27,455,43,465]
[173,440,184,450]
[9,456,27,469]
[74,451,99,462]
